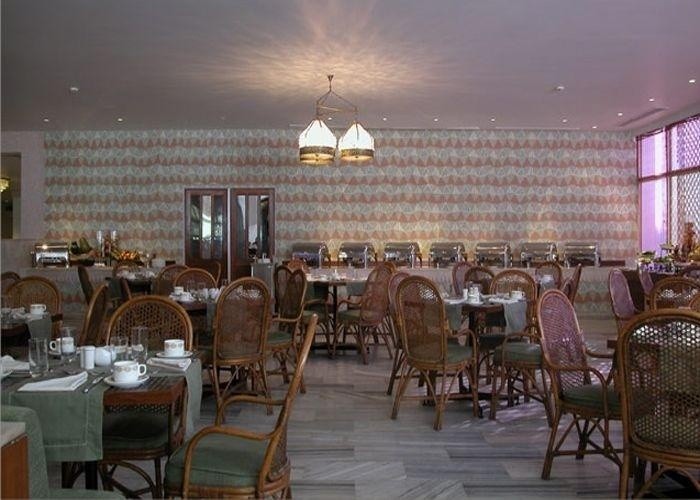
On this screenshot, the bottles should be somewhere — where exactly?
[79,345,95,369]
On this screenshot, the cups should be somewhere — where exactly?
[29,303,46,315]
[28,337,50,380]
[112,360,147,381]
[50,336,76,352]
[164,339,185,356]
[173,286,184,294]
[511,290,526,301]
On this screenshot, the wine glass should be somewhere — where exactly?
[110,336,128,360]
[131,327,147,370]
[1,303,11,325]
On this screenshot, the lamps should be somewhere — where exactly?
[299,75,376,164]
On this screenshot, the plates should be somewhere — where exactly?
[47,348,80,356]
[154,351,194,358]
[102,375,150,389]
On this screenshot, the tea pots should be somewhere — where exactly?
[93,344,115,367]
